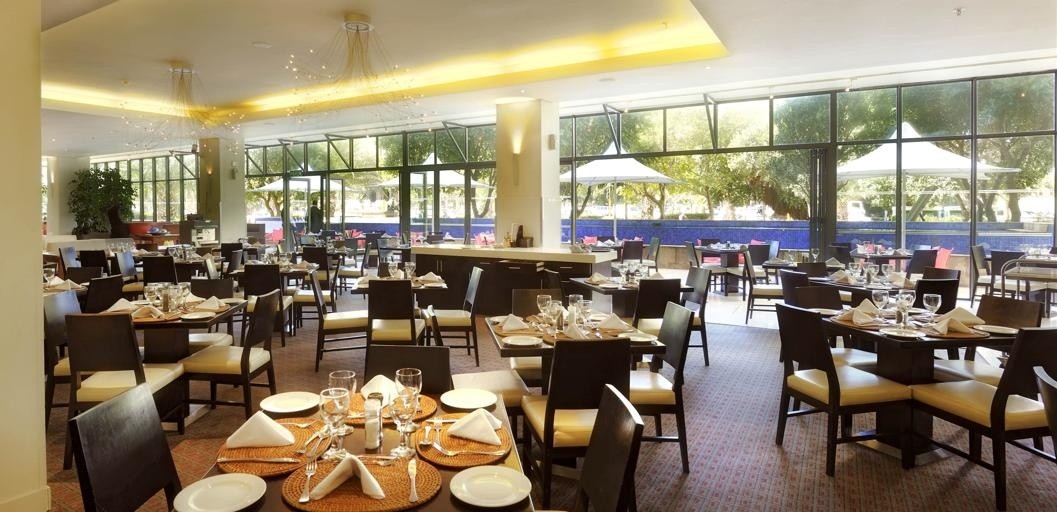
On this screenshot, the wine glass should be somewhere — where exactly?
[871,289,889,320]
[637,264,649,279]
[568,294,583,306]
[811,247,820,263]
[617,264,628,286]
[328,370,357,435]
[895,294,913,329]
[627,261,638,284]
[853,258,865,282]
[319,387,351,460]
[536,294,552,324]
[848,262,859,278]
[882,263,894,285]
[860,262,873,282]
[547,300,563,337]
[395,368,423,429]
[576,300,594,335]
[896,289,917,326]
[143,282,191,309]
[389,388,418,458]
[44,268,55,289]
[922,294,942,327]
[867,264,880,284]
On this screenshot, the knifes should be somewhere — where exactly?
[425,419,460,423]
[217,456,301,464]
[407,457,418,503]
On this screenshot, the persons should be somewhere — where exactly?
[280,198,298,255]
[305,200,322,233]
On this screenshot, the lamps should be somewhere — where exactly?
[509,153,524,188]
[204,172,218,196]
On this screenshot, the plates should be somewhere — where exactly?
[599,284,624,289]
[173,472,267,512]
[808,308,841,316]
[973,324,1019,334]
[259,391,320,413]
[490,316,524,323]
[181,311,216,319]
[590,313,609,321]
[439,388,497,409]
[890,306,929,314]
[864,285,892,290]
[618,332,658,342]
[501,335,543,346]
[220,297,242,303]
[878,327,927,338]
[449,466,532,508]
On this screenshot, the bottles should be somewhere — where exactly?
[630,273,635,283]
[162,290,170,311]
[897,300,907,324]
[368,393,383,442]
[556,306,564,332]
[568,306,576,327]
[866,268,875,284]
[364,399,381,449]
[625,273,629,282]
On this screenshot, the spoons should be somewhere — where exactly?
[305,423,330,457]
[418,426,432,445]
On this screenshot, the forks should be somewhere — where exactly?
[279,421,317,429]
[298,457,317,504]
[433,417,442,446]
[433,441,505,457]
[296,430,319,454]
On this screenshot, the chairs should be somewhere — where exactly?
[67,381,182,512]
[65,267,103,285]
[293,256,342,328]
[508,287,563,389]
[59,246,81,274]
[970,245,992,308]
[824,246,849,268]
[366,279,426,347]
[79,250,111,276]
[321,230,336,241]
[363,343,455,397]
[377,234,390,262]
[308,266,369,373]
[779,268,808,305]
[621,240,643,266]
[44,290,99,438]
[642,236,661,276]
[774,303,913,477]
[912,278,959,315]
[747,245,769,280]
[990,250,1030,301]
[684,241,731,297]
[427,304,531,444]
[421,265,488,368]
[63,313,185,471]
[700,238,721,247]
[596,236,616,243]
[299,234,317,248]
[542,268,568,308]
[178,288,281,433]
[791,284,844,310]
[220,242,245,267]
[342,237,358,268]
[629,300,695,475]
[141,255,179,287]
[631,278,682,330]
[83,273,123,312]
[204,256,221,278]
[224,249,243,275]
[743,251,786,325]
[964,294,1042,362]
[922,265,961,280]
[1031,365,1057,461]
[115,250,138,283]
[909,324,1057,512]
[365,233,382,250]
[302,246,329,270]
[190,278,234,299]
[568,383,646,512]
[905,249,938,274]
[243,264,293,347]
[519,338,632,510]
[338,242,372,296]
[797,262,827,277]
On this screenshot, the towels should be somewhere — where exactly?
[131,305,163,319]
[567,324,588,339]
[597,313,628,330]
[360,374,399,409]
[55,279,81,291]
[359,274,379,283]
[420,271,440,282]
[108,298,138,310]
[447,408,503,446]
[836,307,873,326]
[49,276,65,287]
[589,271,605,282]
[825,257,840,265]
[855,298,880,317]
[831,270,854,284]
[226,410,295,449]
[936,306,985,326]
[894,278,914,289]
[196,296,225,310]
[294,260,311,269]
[309,454,386,500]
[651,272,662,279]
[933,317,973,335]
[180,292,205,302]
[499,313,529,332]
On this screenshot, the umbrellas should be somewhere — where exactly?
[365,152,496,189]
[244,161,360,193]
[836,122,1021,173]
[560,139,689,183]
